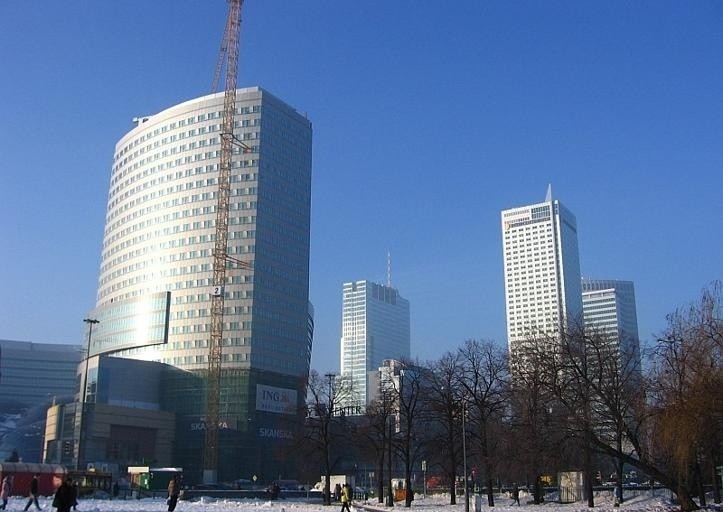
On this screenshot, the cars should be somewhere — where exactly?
[192,479,252,490]
[353,487,367,496]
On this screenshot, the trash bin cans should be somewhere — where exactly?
[472,496,481,512]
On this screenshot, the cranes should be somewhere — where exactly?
[202,0,241,486]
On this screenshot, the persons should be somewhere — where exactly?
[509,486,519,506]
[0,476,9,510]
[70,480,79,512]
[53,478,75,511]
[23,473,42,511]
[340,483,350,512]
[271,483,280,500]
[165,475,179,512]
[333,484,340,501]
[612,484,619,507]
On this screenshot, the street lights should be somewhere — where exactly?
[369,472,374,488]
[253,475,257,486]
[462,396,475,493]
[75,319,104,472]
[325,374,335,412]
[355,464,358,482]
[422,461,426,498]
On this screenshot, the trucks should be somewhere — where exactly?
[310,475,355,497]
